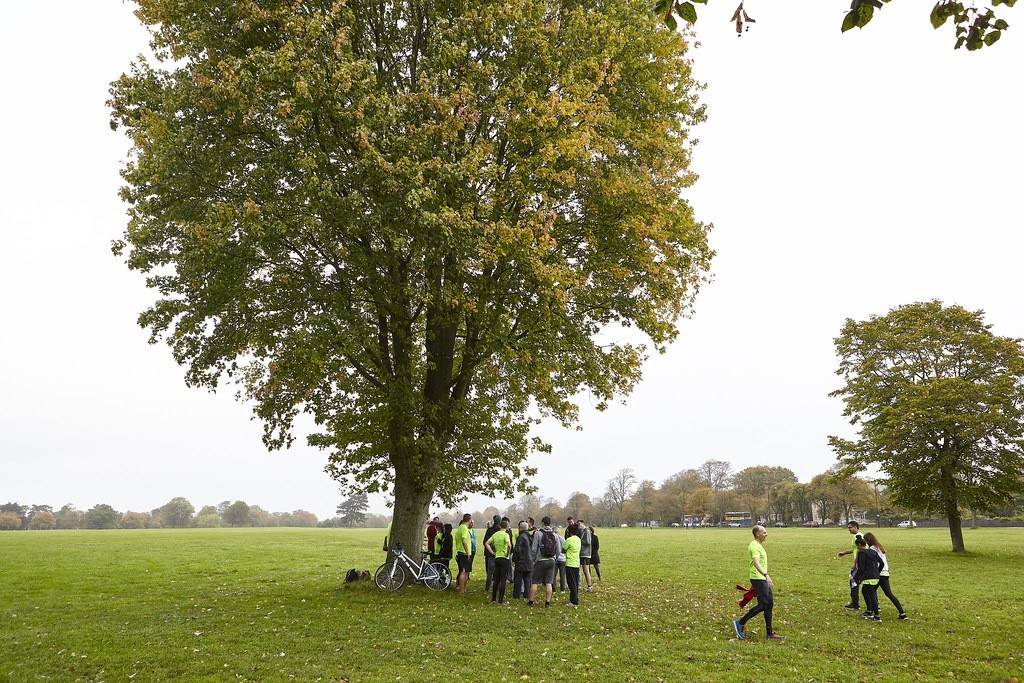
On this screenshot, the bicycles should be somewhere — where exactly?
[375,539,452,591]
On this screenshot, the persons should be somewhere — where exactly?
[422,513,601,607]
[732,526,785,639]
[386,521,392,549]
[862,532,908,620]
[854,535,885,623]
[837,521,869,612]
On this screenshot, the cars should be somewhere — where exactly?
[803,521,820,528]
[897,521,917,528]
[671,523,680,528]
[621,523,629,528]
[773,522,788,527]
[728,521,741,527]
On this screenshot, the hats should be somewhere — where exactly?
[569,524,578,536]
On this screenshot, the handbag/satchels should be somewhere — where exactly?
[360,570,370,580]
[346,568,359,582]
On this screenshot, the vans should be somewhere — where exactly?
[839,520,852,526]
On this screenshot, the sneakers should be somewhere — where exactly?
[878,607,881,611]
[872,615,882,623]
[733,620,745,639]
[767,633,785,639]
[862,611,874,618]
[844,603,860,610]
[897,613,908,621]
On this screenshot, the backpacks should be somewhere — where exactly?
[538,528,556,558]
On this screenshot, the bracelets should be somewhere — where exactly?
[764,573,768,577]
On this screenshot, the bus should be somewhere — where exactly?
[683,514,713,527]
[725,512,751,523]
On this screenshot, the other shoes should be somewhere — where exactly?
[545,605,552,609]
[498,602,506,606]
[587,587,592,592]
[527,601,534,606]
[490,601,496,604]
[565,603,578,610]
